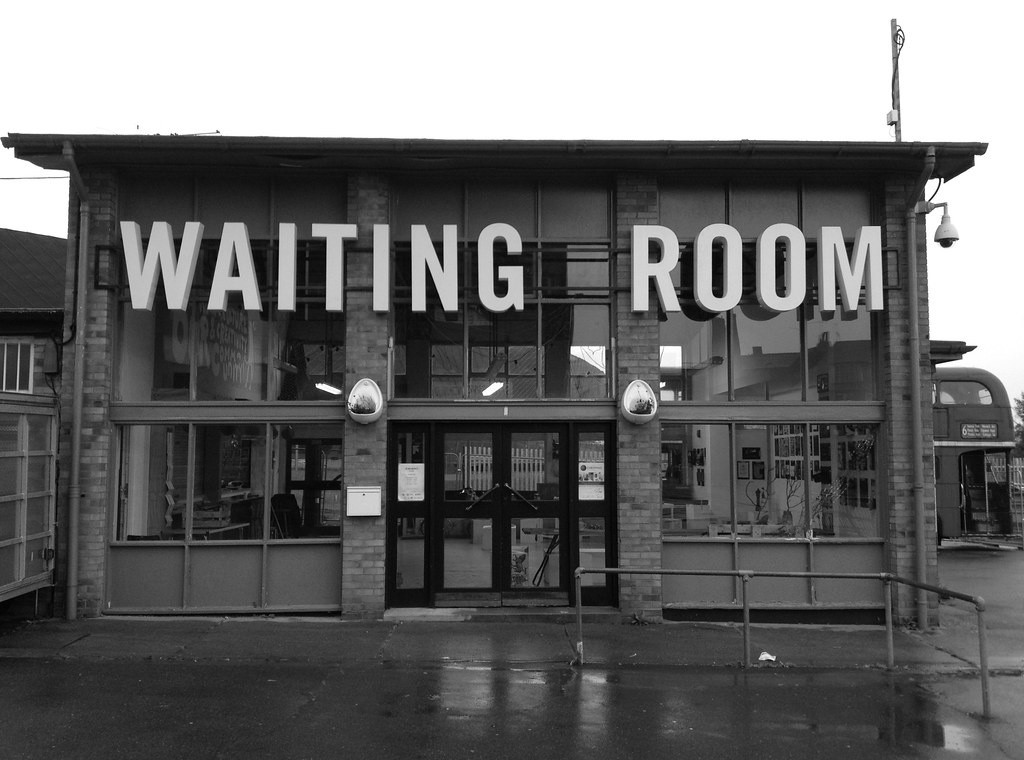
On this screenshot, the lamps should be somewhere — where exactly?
[314,341,343,395]
[482,312,507,397]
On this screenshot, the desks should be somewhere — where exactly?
[163,523,250,541]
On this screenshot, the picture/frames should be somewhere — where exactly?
[742,447,761,459]
[737,461,750,479]
[751,461,765,480]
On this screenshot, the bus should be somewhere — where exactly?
[931,368,1015,547]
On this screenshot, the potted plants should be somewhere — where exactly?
[620,380,657,424]
[347,379,384,424]
